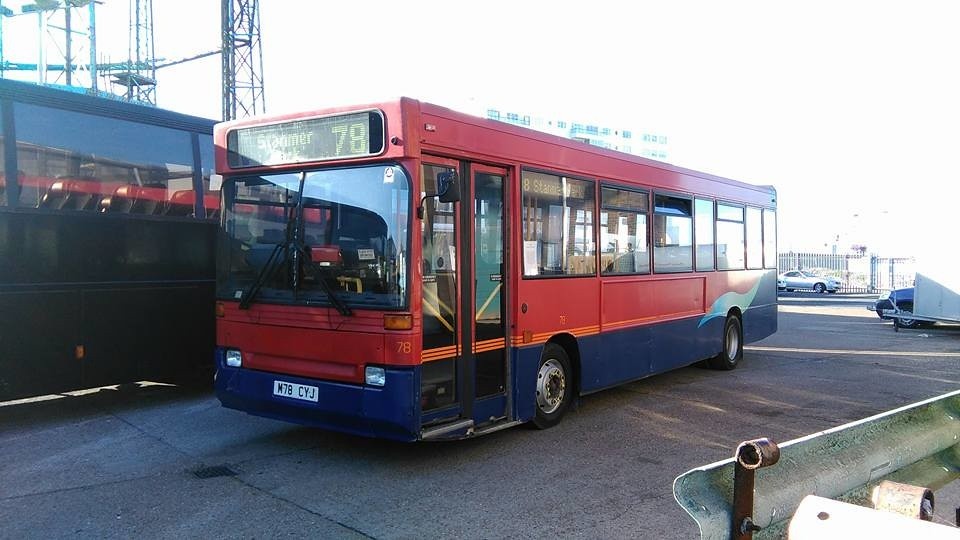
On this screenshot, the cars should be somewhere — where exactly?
[779,268,843,294]
[866,283,938,329]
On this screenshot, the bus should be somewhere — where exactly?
[0,74,235,409]
[207,94,780,446]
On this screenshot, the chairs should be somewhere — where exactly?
[0,169,228,217]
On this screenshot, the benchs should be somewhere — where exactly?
[568,243,728,274]
